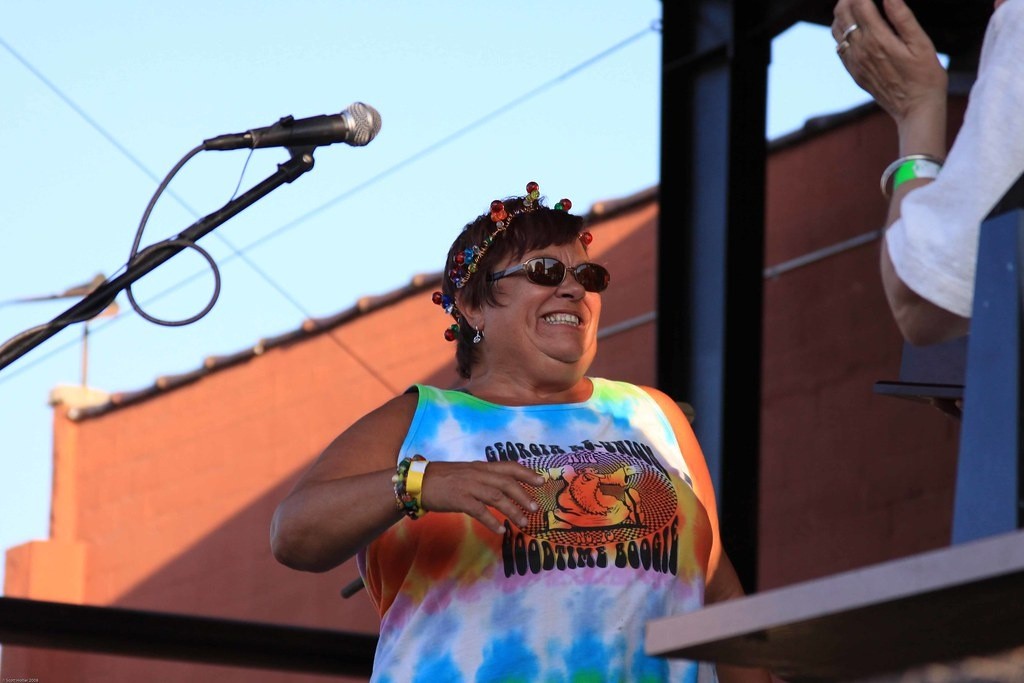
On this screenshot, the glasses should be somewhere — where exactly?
[491,257,611,293]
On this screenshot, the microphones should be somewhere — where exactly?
[204,102,383,150]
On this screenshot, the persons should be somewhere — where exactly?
[830,0,1024,345]
[270,182,747,683]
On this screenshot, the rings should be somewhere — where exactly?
[842,24,860,38]
[837,40,849,53]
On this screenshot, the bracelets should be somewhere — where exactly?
[391,454,431,520]
[880,154,945,199]
